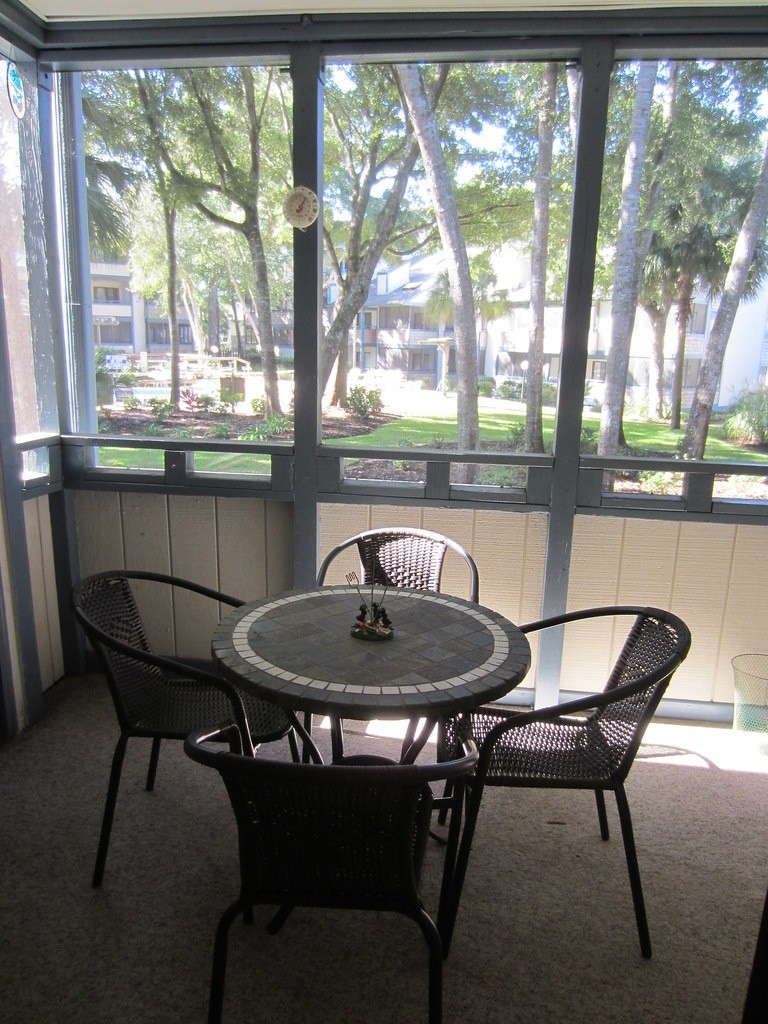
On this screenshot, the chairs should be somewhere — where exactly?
[178,716,481,1024]
[434,604,692,963]
[68,565,315,895]
[297,525,482,822]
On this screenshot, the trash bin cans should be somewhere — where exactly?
[730,652,768,754]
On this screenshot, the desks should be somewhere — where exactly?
[211,581,530,842]
